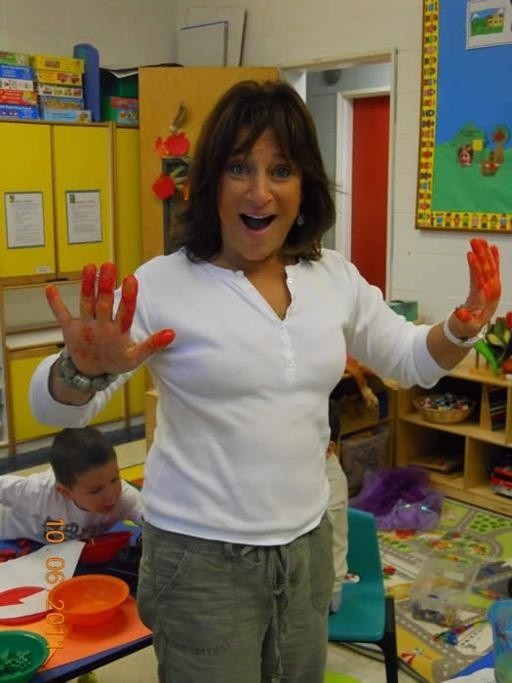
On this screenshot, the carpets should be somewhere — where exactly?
[330,493,512,683]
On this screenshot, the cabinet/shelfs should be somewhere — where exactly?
[336,351,512,518]
[2,118,148,470]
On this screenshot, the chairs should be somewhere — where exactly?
[328,507,397,683]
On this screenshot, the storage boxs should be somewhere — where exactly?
[0,49,139,127]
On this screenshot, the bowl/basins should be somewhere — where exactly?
[74,528,136,566]
[0,629,52,683]
[46,571,131,627]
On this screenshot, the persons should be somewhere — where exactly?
[326,402,348,612]
[29,81,501,683]
[457,143,473,166]
[0,426,142,546]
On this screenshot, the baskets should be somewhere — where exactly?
[413,393,474,424]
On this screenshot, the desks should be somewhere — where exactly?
[1,537,155,682]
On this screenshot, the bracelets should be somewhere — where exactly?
[58,343,119,392]
[443,304,488,347]
